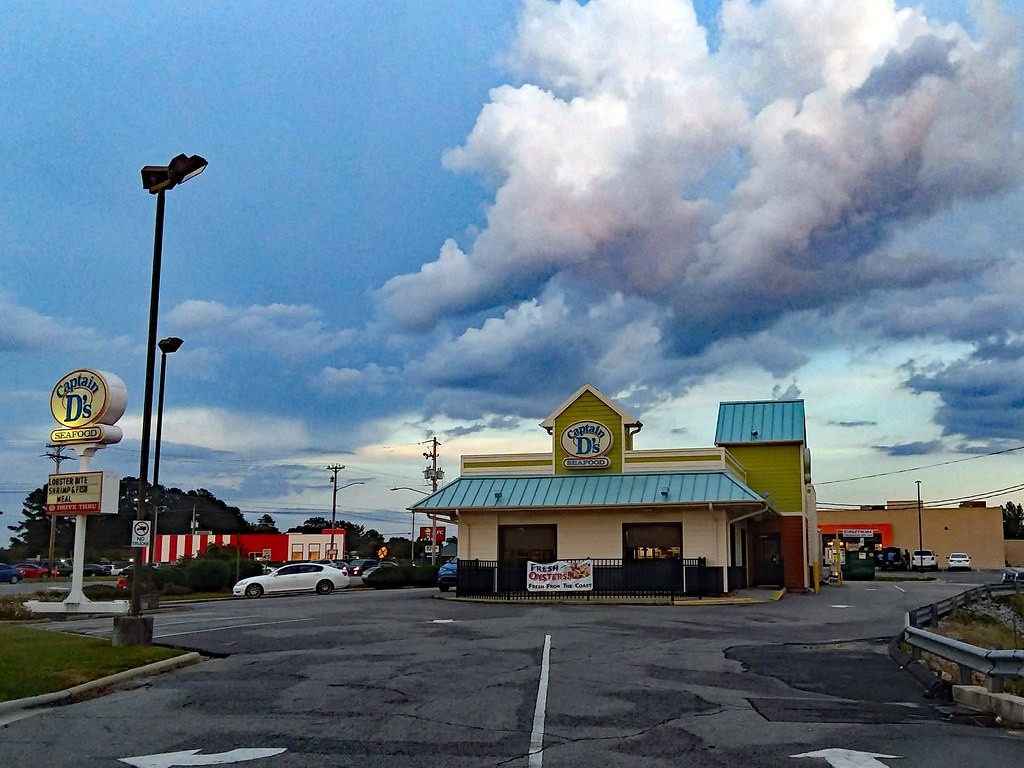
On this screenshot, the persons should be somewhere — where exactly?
[902,548,910,570]
[877,550,896,571]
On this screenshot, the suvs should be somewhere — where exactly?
[881,546,910,571]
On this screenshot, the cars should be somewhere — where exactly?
[11,563,60,579]
[438,555,457,592]
[35,562,73,577]
[310,559,380,576]
[83,564,111,577]
[0,563,24,584]
[911,550,939,572]
[946,552,972,571]
[261,564,277,572]
[233,562,351,600]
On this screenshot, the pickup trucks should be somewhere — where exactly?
[102,565,123,576]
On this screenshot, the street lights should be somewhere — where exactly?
[390,487,436,565]
[111,152,209,649]
[139,337,185,610]
[152,506,166,563]
[914,480,922,573]
[330,482,365,559]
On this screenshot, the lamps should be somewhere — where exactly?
[760,492,769,499]
[751,430,758,438]
[771,499,776,506]
[494,488,503,501]
[660,486,669,496]
